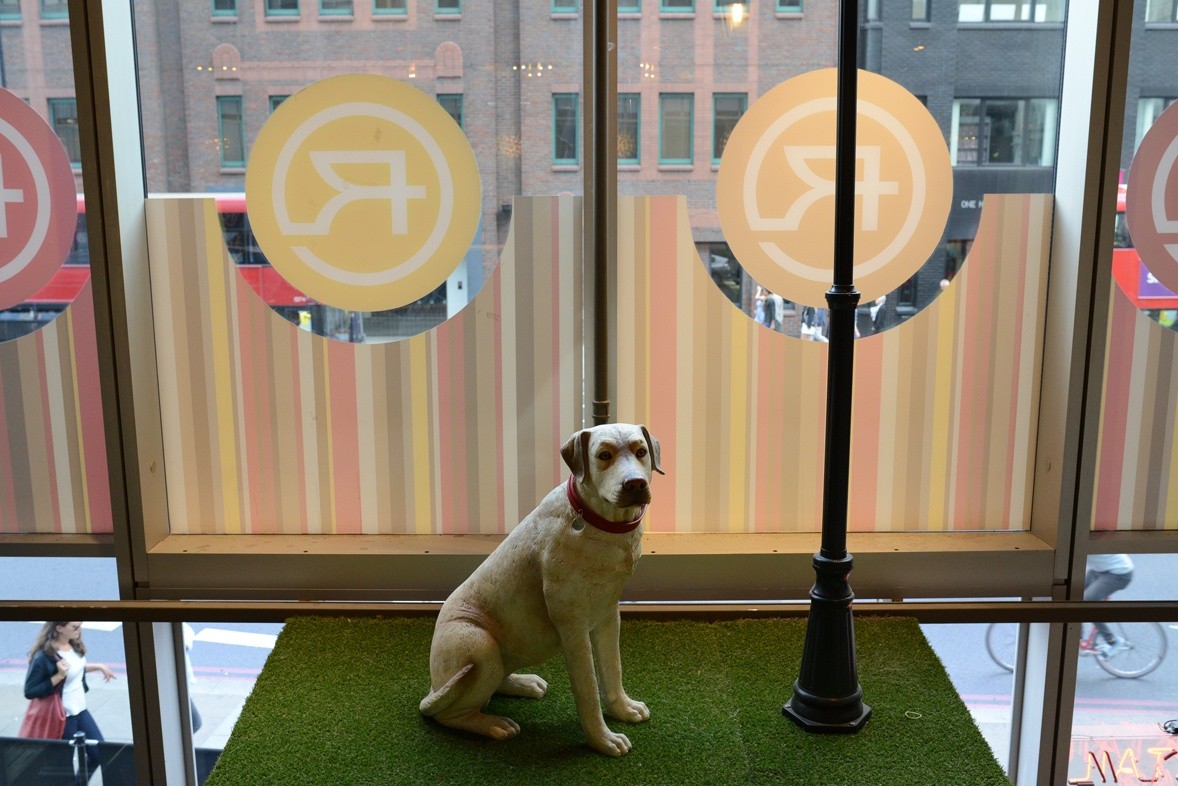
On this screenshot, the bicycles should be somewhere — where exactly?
[985,597,1170,681]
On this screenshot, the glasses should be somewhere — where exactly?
[67,622,81,631]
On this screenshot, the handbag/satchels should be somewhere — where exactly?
[18,694,67,739]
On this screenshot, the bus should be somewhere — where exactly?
[0,192,447,343]
[1111,183,1178,330]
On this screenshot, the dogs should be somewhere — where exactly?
[418,422,668,757]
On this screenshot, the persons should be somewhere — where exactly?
[940,279,950,290]
[1080,553,1133,660]
[23,621,118,742]
[854,308,860,339]
[870,295,887,335]
[182,622,202,734]
[752,285,784,332]
[801,306,829,342]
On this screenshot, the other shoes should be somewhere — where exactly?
[1099,638,1126,659]
